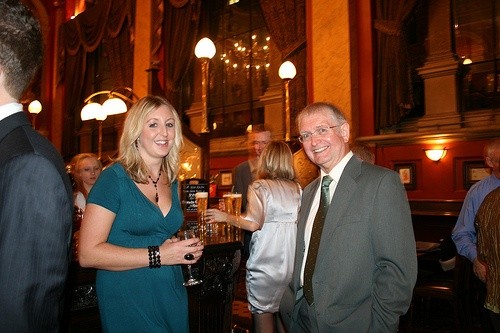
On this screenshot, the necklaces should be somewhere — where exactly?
[149,173,161,203]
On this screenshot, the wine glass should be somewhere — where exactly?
[177,230,202,286]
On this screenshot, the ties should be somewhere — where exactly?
[303,175,332,306]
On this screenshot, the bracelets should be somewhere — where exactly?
[148,246,161,268]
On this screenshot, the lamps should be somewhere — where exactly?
[81,86,144,124]
[194,36,217,133]
[278,61,298,141]
[423,149,452,161]
[28,99,42,131]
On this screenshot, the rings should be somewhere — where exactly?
[184,253,194,260]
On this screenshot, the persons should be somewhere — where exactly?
[71,153,102,264]
[279,103,418,333]
[0,0,73,333]
[231,126,272,214]
[78,96,204,333]
[451,137,500,333]
[202,140,303,333]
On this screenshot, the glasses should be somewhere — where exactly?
[298,122,345,143]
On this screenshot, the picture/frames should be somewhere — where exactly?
[217,169,236,190]
[464,159,494,188]
[392,162,417,190]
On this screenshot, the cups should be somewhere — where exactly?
[195,192,208,216]
[223,194,242,217]
